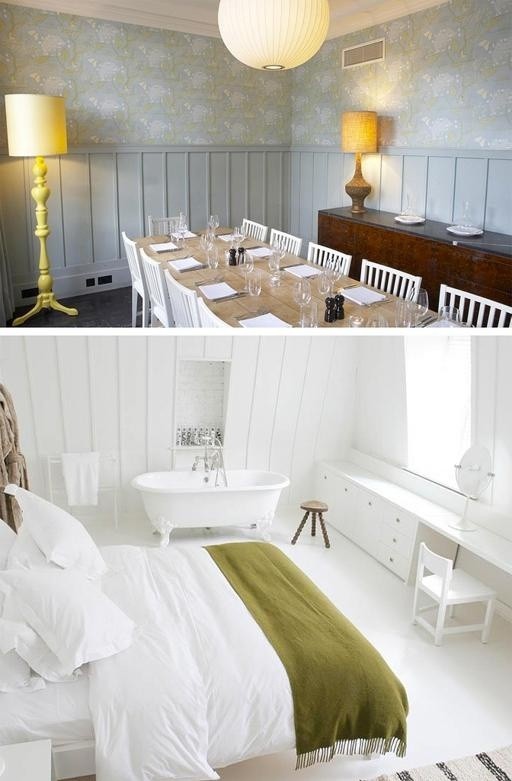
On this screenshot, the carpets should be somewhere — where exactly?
[369,745,512,781]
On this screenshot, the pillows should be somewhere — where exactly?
[6,567,136,673]
[4,484,106,578]
[1,581,62,682]
[6,523,62,573]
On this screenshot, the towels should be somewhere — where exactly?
[61,452,99,506]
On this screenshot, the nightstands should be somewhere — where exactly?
[0,739,52,781]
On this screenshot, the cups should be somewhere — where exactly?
[394,300,411,328]
[269,251,280,270]
[230,236,241,248]
[317,271,330,293]
[208,247,219,269]
[300,301,318,328]
[267,271,281,287]
[249,269,261,297]
[349,313,366,327]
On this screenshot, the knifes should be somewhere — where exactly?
[215,295,246,303]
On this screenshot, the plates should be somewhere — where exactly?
[446,226,483,237]
[394,216,426,225]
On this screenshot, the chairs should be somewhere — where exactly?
[122,205,512,328]
[412,542,496,647]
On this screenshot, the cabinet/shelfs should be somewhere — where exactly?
[314,460,511,649]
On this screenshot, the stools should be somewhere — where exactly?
[291,502,330,548]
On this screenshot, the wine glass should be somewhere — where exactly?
[450,322,475,328]
[325,259,343,294]
[208,214,220,234]
[178,222,187,244]
[405,288,428,316]
[200,234,215,265]
[239,253,254,290]
[365,313,388,327]
[437,306,460,328]
[272,240,286,259]
[292,281,312,325]
[234,225,244,242]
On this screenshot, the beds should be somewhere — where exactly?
[0,519,407,781]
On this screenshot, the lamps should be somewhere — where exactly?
[218,0,330,71]
[4,94,79,326]
[342,111,377,213]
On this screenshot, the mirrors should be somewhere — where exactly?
[448,444,495,531]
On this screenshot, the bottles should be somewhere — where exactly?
[204,428,209,445]
[199,428,204,445]
[216,428,221,446]
[238,245,245,265]
[187,428,192,446]
[229,246,236,266]
[192,429,198,445]
[210,429,216,446]
[181,428,187,446]
[324,295,336,322]
[335,292,345,319]
[177,428,181,445]
